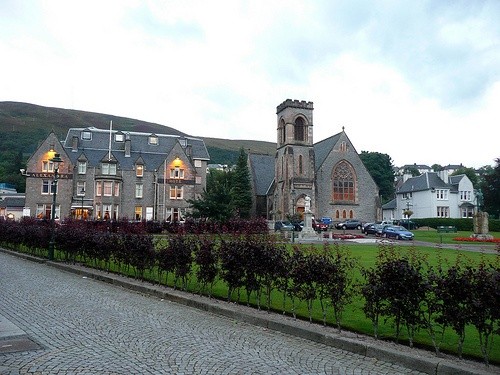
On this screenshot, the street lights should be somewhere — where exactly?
[47,153,64,260]
[80,188,86,220]
[290,189,297,244]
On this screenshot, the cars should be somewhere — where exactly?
[275,220,295,233]
[381,224,415,241]
[292,217,390,237]
